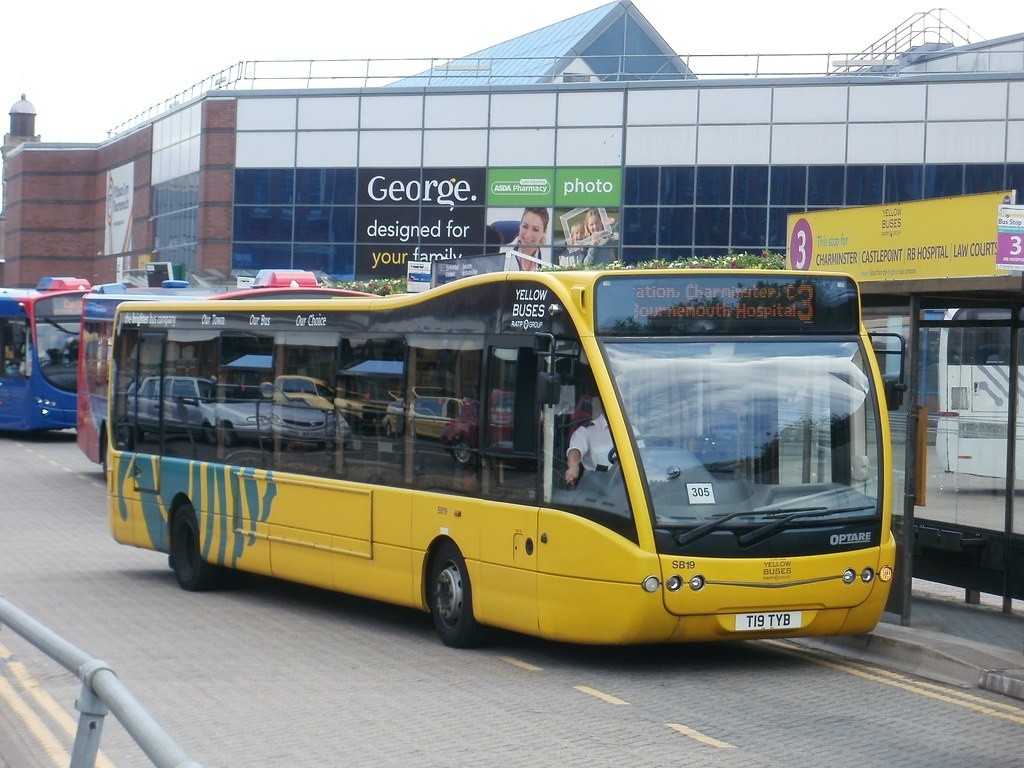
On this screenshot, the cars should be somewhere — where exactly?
[332,370,400,435]
[381,385,474,439]
[570,394,604,446]
[259,375,339,414]
[122,374,337,450]
[439,389,541,470]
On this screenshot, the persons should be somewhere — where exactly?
[560,208,618,268]
[564,402,644,485]
[498,207,551,273]
[19,339,52,377]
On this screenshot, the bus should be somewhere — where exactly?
[917,309,946,415]
[77,271,384,482]
[107,268,907,649]
[936,308,1024,492]
[0,275,94,432]
